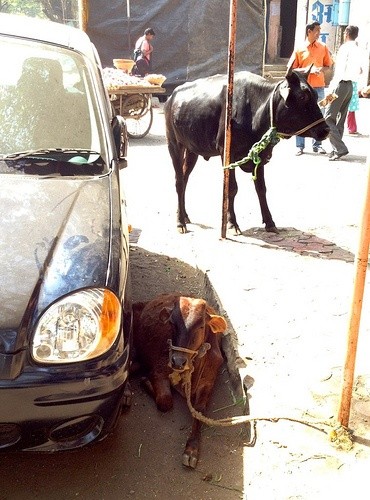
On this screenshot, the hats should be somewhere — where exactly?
[143,28,156,35]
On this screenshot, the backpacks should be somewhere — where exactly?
[133,40,153,76]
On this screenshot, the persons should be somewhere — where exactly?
[325,25,362,161]
[134,28,155,75]
[286,21,326,156]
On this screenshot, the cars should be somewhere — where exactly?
[0,11,129,458]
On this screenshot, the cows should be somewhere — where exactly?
[162,57,333,236]
[125,292,227,471]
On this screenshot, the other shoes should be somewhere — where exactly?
[348,132,362,137]
[313,146,326,154]
[295,147,304,156]
[328,149,349,161]
